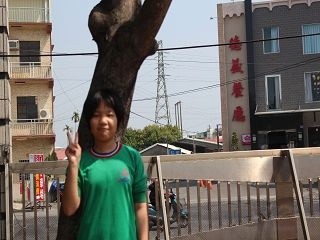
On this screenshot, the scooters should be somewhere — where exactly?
[142,187,193,228]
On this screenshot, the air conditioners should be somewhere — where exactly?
[40,109,51,119]
[8,40,19,50]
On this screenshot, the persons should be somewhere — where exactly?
[147,179,175,215]
[61,90,150,240]
